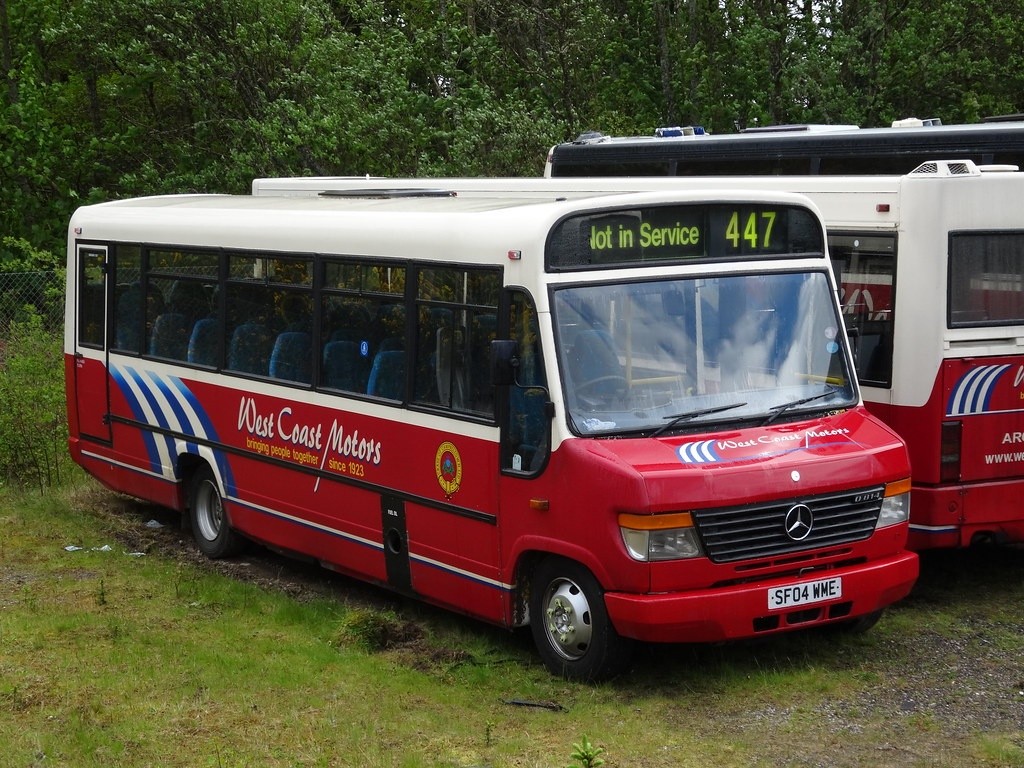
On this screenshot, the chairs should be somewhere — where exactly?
[86,271,647,425]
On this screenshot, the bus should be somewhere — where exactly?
[544,116,1024,178]
[65,191,921,682]
[250,159,1024,555]
